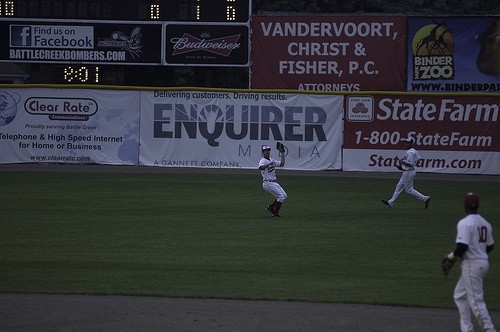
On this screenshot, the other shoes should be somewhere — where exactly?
[424,196,431,209]
[381,199,391,207]
[275,211,281,218]
[264,206,275,217]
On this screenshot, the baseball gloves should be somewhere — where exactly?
[440,255,454,275]
[276,141,286,153]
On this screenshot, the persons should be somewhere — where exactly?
[258,141,288,217]
[381,137,431,209]
[441,192,497,332]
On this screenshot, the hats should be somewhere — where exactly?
[403,138,413,144]
[262,145,272,153]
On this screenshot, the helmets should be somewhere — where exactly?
[463,191,480,205]
